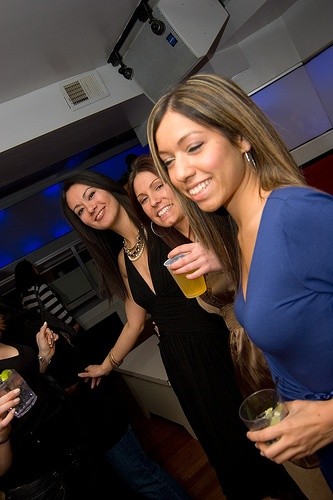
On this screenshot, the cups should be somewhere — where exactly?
[1,369,37,418]
[164,252,207,298]
[238,388,289,447]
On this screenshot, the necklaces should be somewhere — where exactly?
[122,229,146,261]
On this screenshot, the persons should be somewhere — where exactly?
[62,169,310,500]
[14,259,82,336]
[147,76,333,493]
[0,315,140,499]
[129,156,323,468]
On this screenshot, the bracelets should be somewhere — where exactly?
[0,437,11,444]
[38,356,51,365]
[107,350,124,369]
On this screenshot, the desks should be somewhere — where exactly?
[111,334,199,441]
[76,293,127,331]
